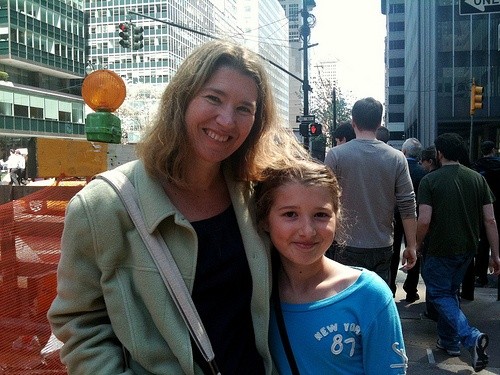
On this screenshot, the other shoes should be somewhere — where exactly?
[459,291,475,300]
[406,293,419,303]
[475,276,488,288]
[424,312,439,322]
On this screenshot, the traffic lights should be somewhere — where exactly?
[118,22,131,49]
[471,85,484,111]
[307,122,322,137]
[130,23,144,52]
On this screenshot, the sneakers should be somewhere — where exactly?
[469,332,488,373]
[435,338,461,356]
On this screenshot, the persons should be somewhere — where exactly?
[326,98,500,373]
[249,157,408,375]
[3,149,28,187]
[48,38,309,375]
